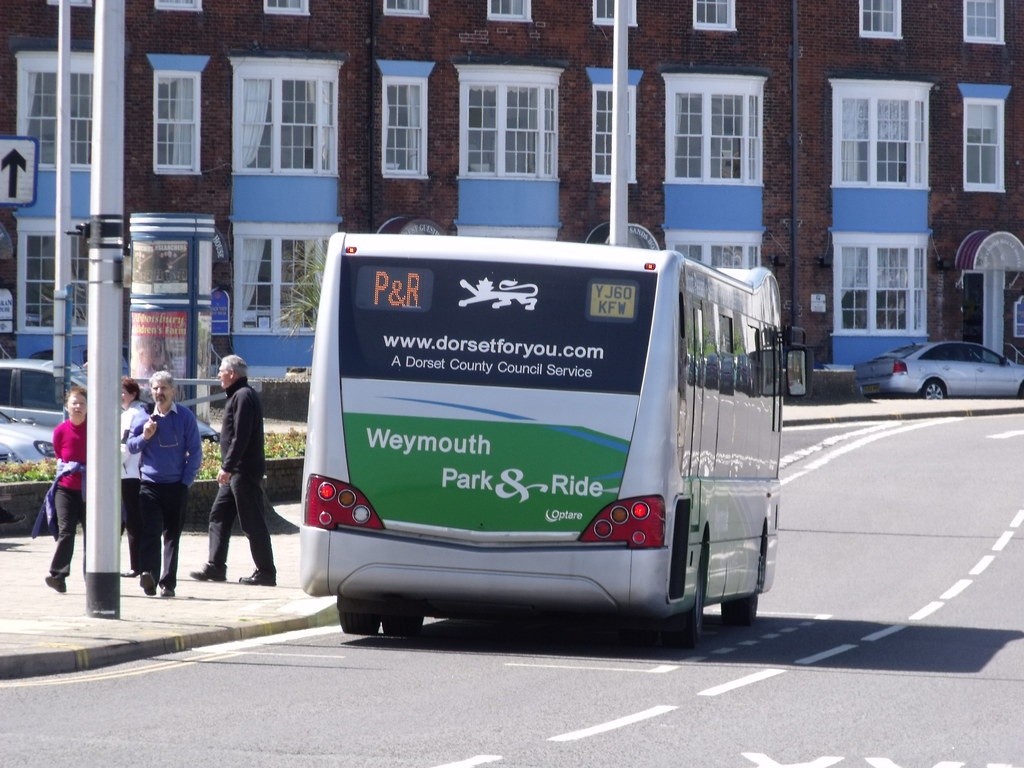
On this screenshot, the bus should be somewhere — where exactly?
[297,235,810,647]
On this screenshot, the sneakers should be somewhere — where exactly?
[239,571,277,587]
[190,569,226,581]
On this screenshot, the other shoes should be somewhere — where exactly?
[140,572,156,595]
[161,586,175,597]
[119,568,143,577]
[45,575,66,593]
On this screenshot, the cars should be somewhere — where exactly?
[853,338,1024,399]
[0,360,219,464]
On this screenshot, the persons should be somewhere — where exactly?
[126,371,202,597]
[190,355,279,587]
[46,386,88,593]
[119,379,147,573]
